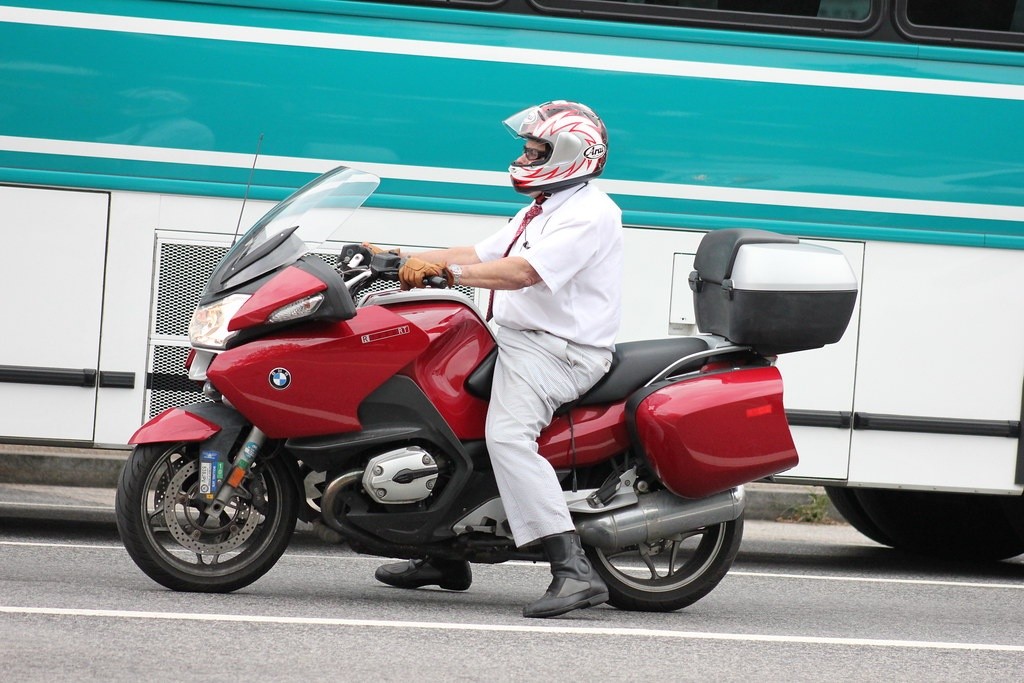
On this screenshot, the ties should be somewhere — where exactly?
[485,201,542,322]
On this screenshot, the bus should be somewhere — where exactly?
[1,0,1024,562]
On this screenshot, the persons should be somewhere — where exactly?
[357,99,621,615]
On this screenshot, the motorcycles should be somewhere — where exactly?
[115,165,858,613]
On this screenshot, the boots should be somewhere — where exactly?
[523,530,609,618]
[374,545,471,592]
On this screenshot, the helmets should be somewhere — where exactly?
[508,100,608,192]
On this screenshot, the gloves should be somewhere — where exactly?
[360,241,401,261]
[398,258,454,291]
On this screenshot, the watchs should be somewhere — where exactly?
[449,264,462,285]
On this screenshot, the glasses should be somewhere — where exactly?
[522,145,549,162]
